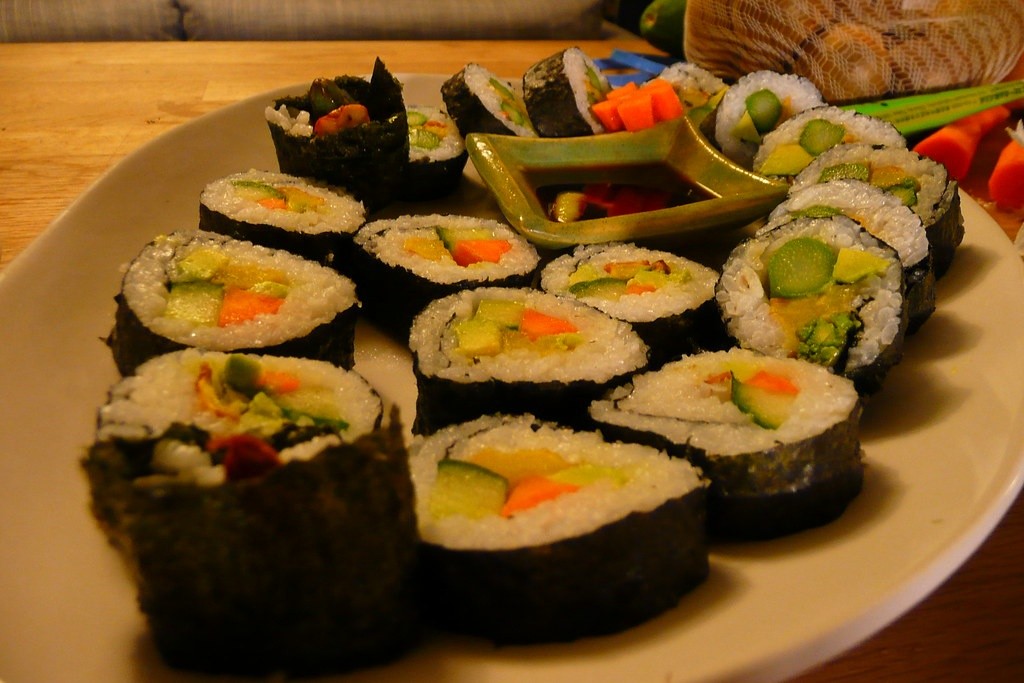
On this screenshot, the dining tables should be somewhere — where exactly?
[0,22,1024,682]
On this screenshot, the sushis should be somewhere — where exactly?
[79,47,969,681]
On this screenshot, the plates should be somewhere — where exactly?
[1,63,1024,681]
[460,108,791,250]
